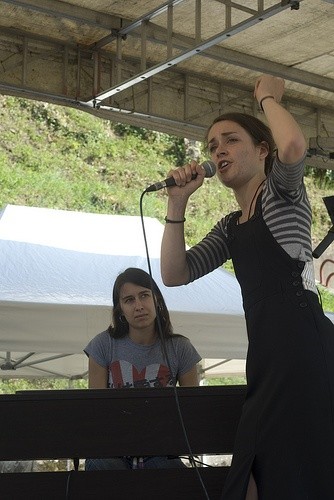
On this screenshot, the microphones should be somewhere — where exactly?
[146,160,217,193]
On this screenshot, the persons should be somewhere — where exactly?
[160,75,334,500]
[82,267,202,469]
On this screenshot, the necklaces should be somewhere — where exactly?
[237,177,267,225]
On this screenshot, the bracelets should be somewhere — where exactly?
[164,216,186,223]
[260,96,274,112]
